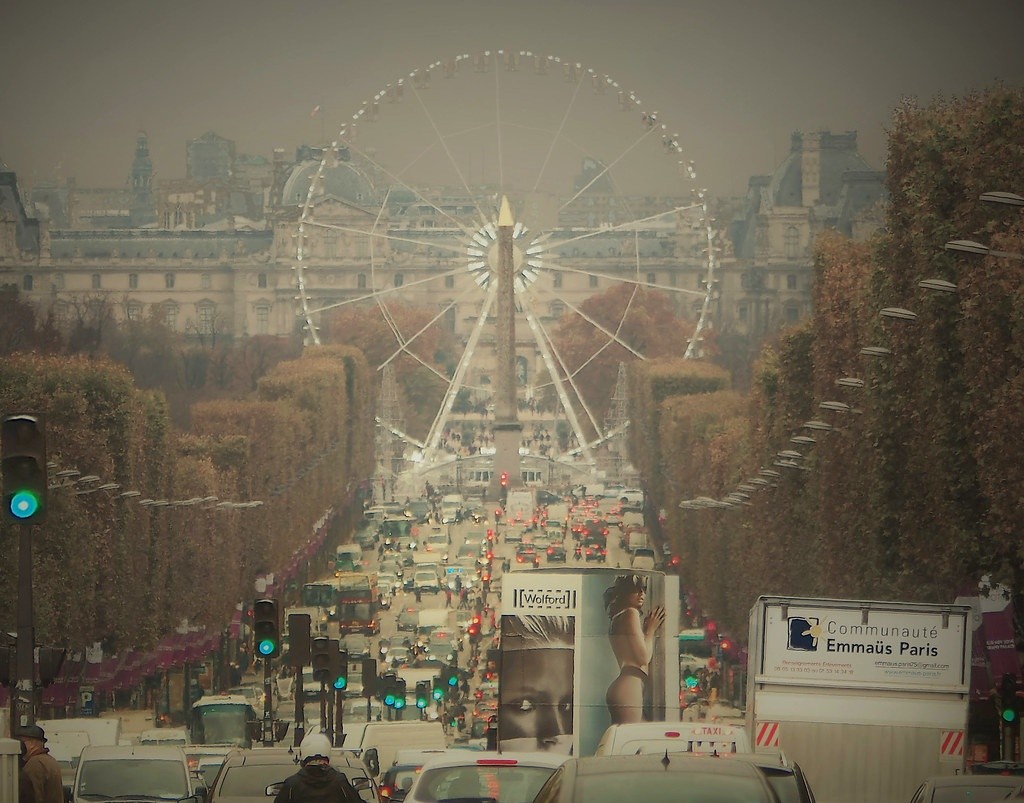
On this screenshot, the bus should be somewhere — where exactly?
[303,578,335,619]
[335,574,381,635]
[187,695,258,748]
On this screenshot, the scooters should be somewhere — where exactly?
[574,548,581,560]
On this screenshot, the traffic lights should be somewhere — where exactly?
[468,628,476,636]
[501,481,507,486]
[495,510,500,522]
[0,412,48,527]
[393,678,406,709]
[472,616,481,624]
[482,577,488,581]
[433,675,445,700]
[1001,673,1018,726]
[487,553,492,559]
[445,665,458,696]
[383,674,396,706]
[415,682,427,708]
[253,598,280,658]
[247,717,262,740]
[288,614,310,666]
[487,530,492,535]
[311,635,331,681]
[274,720,290,743]
[332,650,348,690]
[487,536,493,541]
[330,640,338,681]
[362,658,377,695]
[501,474,506,480]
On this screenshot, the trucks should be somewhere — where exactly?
[745,595,974,803]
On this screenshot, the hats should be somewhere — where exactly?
[14,725,47,743]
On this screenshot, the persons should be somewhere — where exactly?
[444,575,489,611]
[500,614,576,759]
[599,573,665,723]
[273,734,368,803]
[15,725,65,803]
[401,627,431,656]
[683,665,723,695]
[437,616,482,734]
[376,544,384,561]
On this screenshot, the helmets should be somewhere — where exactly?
[299,734,331,767]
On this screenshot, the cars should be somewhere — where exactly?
[28,718,1024,802]
[304,484,654,738]
